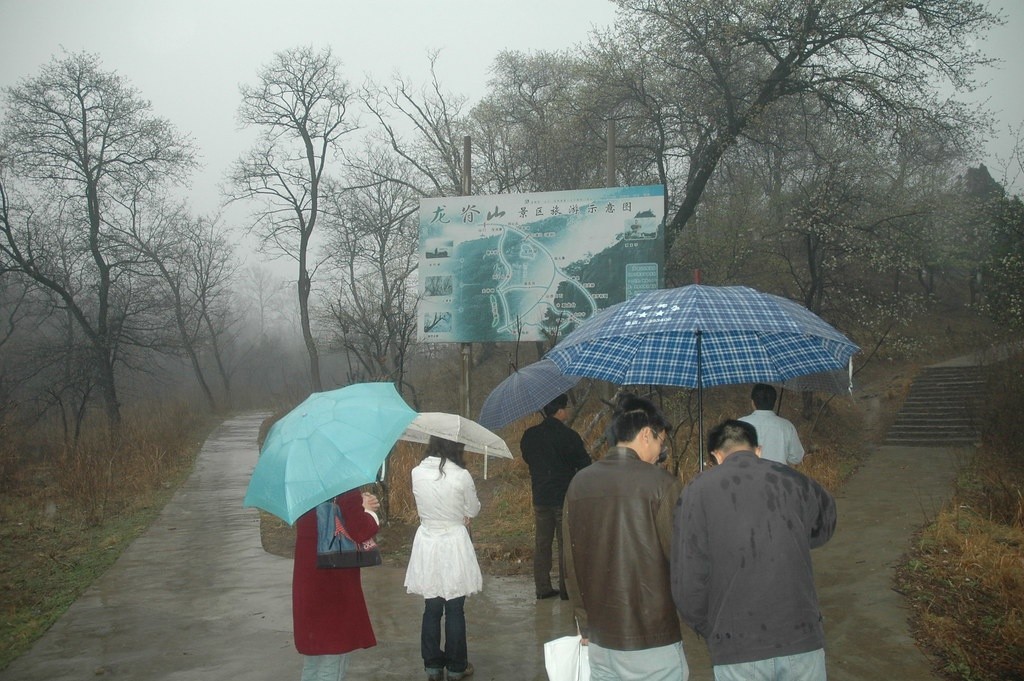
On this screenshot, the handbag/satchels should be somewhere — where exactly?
[315,502,382,569]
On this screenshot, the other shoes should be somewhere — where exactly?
[448,663,474,680]
[537,589,560,599]
[429,672,443,681]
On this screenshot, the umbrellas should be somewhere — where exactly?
[763,368,854,416]
[479,359,583,430]
[541,270,865,472]
[244,383,422,527]
[398,412,514,479]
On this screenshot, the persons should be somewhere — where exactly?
[739,382,805,466]
[293,488,380,681]
[670,420,837,681]
[404,435,481,681]
[562,399,689,681]
[521,393,592,600]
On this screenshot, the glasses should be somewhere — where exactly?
[651,429,668,453]
